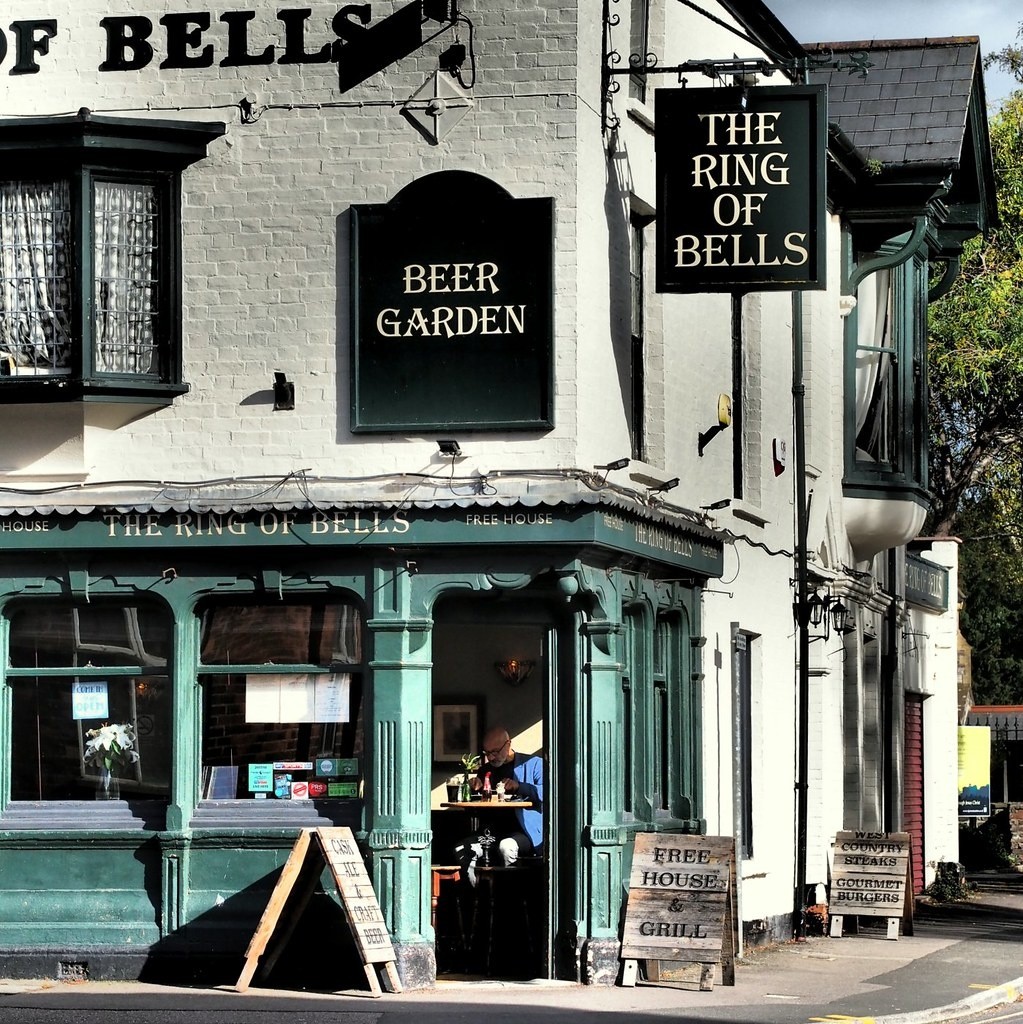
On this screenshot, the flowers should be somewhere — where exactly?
[82,723,140,770]
[461,752,482,771]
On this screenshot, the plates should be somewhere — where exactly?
[491,795,513,802]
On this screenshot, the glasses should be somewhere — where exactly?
[483,740,511,756]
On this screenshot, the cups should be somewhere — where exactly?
[445,777,459,803]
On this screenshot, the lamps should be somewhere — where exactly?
[646,477,679,492]
[699,498,730,510]
[788,578,823,629]
[435,439,461,456]
[593,457,630,470]
[822,593,848,636]
[497,660,536,685]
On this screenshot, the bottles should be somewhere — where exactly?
[482,776,492,802]
[496,781,506,802]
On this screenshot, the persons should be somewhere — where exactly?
[441,726,543,887]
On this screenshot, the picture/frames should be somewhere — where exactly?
[430,691,487,771]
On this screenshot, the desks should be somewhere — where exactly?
[440,801,531,865]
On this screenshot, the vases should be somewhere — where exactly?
[95,756,120,800]
[461,771,471,802]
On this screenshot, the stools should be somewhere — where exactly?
[431,866,465,945]
[466,864,527,978]
